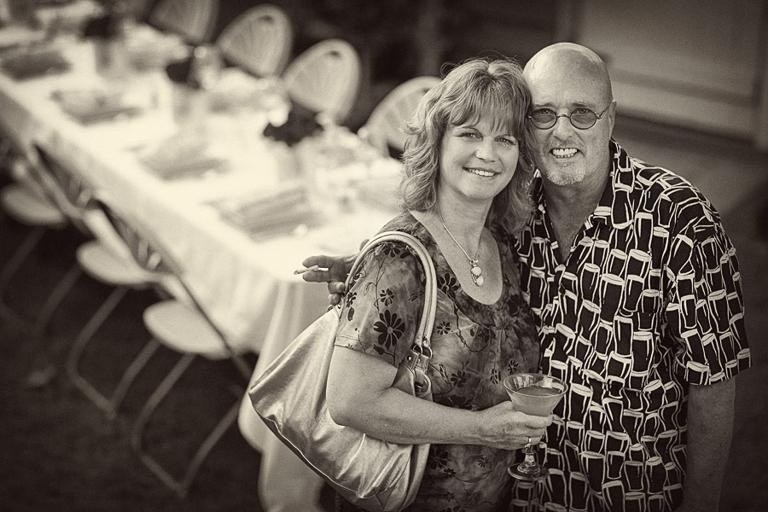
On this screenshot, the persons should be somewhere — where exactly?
[298,40,753,511]
[311,51,554,511]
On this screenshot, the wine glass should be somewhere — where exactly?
[502,373,570,482]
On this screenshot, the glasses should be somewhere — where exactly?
[523,106,608,130]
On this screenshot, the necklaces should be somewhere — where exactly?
[438,205,491,292]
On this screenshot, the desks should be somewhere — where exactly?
[1,0,425,399]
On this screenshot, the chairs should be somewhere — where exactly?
[365,74,442,160]
[133,263,269,492]
[0,137,93,367]
[67,202,170,418]
[215,5,290,84]
[281,39,363,124]
[154,2,214,43]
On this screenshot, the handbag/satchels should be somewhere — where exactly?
[248,232,437,511]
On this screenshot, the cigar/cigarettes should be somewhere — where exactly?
[293,264,321,275]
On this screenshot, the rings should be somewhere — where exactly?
[527,438,533,446]
